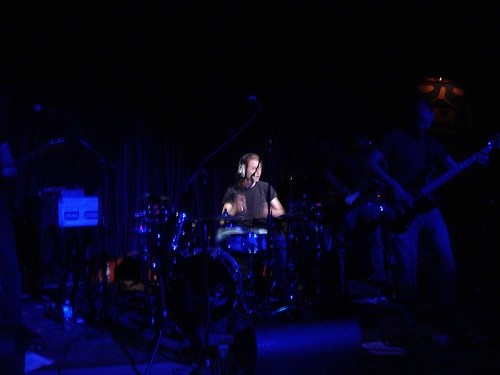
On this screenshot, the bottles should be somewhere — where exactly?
[223,209,228,217]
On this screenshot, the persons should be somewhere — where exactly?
[367,97,488,324]
[220,153,286,226]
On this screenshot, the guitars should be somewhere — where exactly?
[374,141,495,235]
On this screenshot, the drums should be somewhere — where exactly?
[147,210,192,260]
[134,209,169,234]
[198,214,242,246]
[226,224,271,256]
[170,247,242,325]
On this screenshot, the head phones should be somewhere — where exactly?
[238,153,252,178]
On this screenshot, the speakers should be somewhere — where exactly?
[236,318,362,374]
